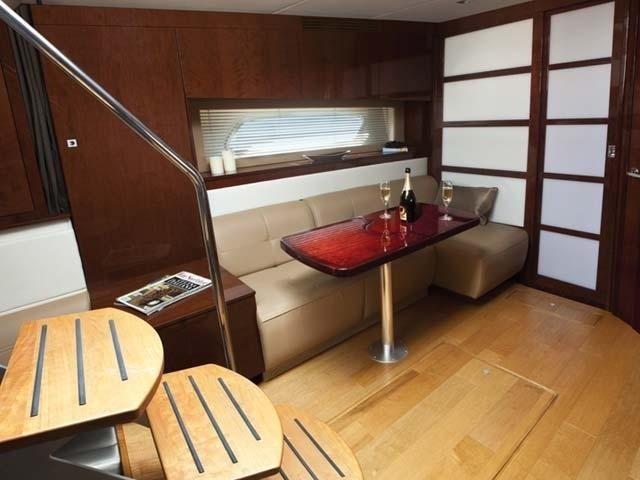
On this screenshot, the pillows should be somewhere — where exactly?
[432,180,499,228]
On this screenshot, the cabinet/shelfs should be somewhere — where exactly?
[88,258,264,383]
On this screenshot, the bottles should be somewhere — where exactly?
[399,222,414,247]
[399,167,417,222]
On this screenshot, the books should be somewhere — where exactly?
[113,274,172,312]
[116,271,213,317]
[383,145,409,154]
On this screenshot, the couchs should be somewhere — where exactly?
[210,174,531,384]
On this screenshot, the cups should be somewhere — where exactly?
[210,156,224,175]
[222,149,237,175]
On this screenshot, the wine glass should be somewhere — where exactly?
[439,181,455,222]
[378,179,392,220]
[379,220,392,250]
